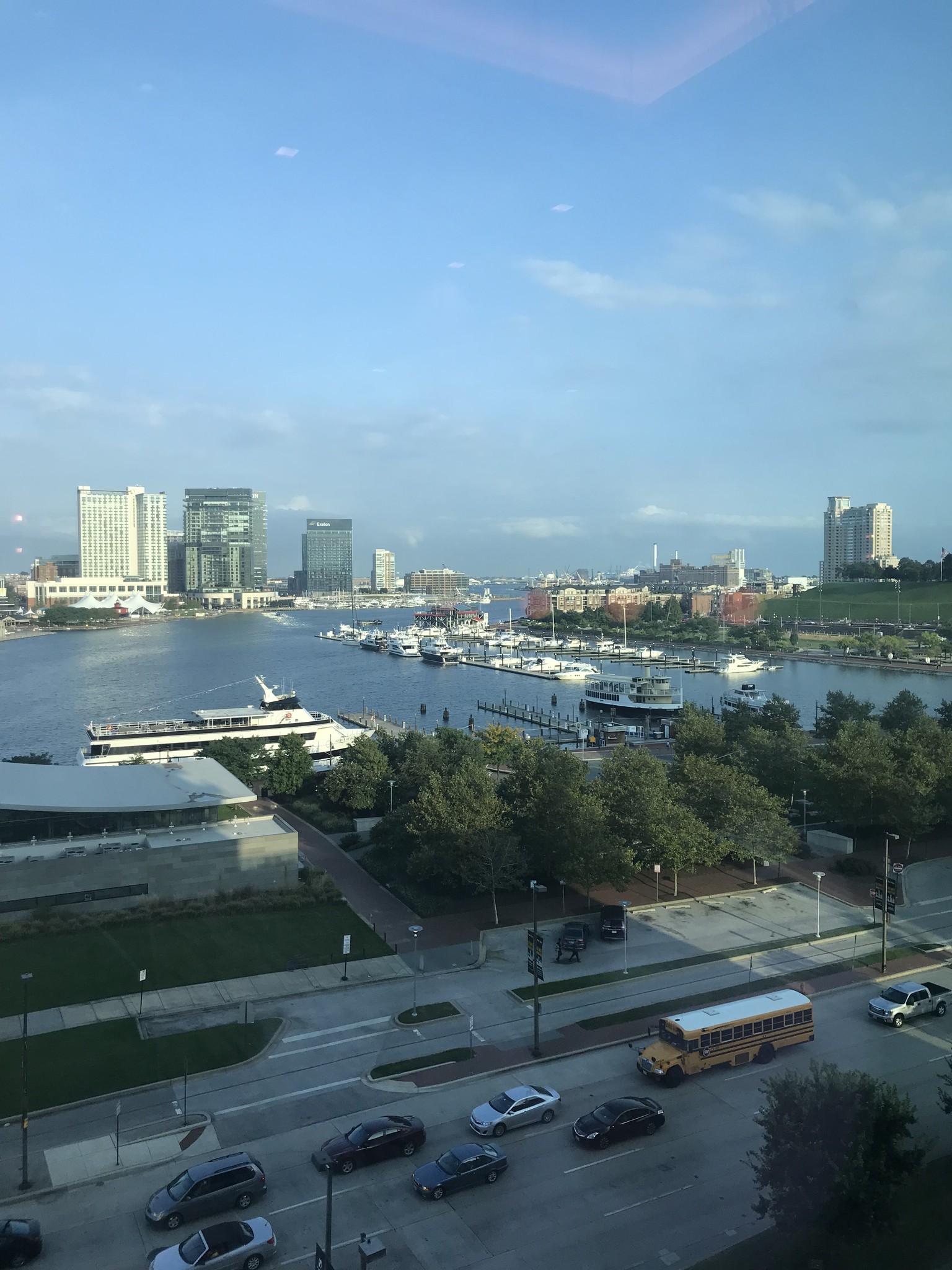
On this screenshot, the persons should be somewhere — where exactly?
[556,937,563,962]
[570,940,582,962]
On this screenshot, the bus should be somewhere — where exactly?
[628,989,814,1088]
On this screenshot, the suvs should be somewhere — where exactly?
[145,1152,266,1230]
[600,905,627,942]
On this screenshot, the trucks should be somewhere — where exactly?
[0,842,123,866]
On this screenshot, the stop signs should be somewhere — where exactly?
[893,863,903,874]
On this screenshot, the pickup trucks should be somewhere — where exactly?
[867,981,952,1028]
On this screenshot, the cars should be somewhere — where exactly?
[562,921,589,949]
[149,1216,277,1270]
[320,1115,425,1175]
[411,1143,508,1200]
[572,1097,664,1150]
[469,1085,562,1138]
[0,1219,43,1268]
[682,618,930,626]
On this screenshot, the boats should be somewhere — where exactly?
[342,572,768,679]
[76,674,375,775]
[720,681,776,717]
[585,659,684,717]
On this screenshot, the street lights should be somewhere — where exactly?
[409,833,900,1058]
[358,1238,387,1270]
